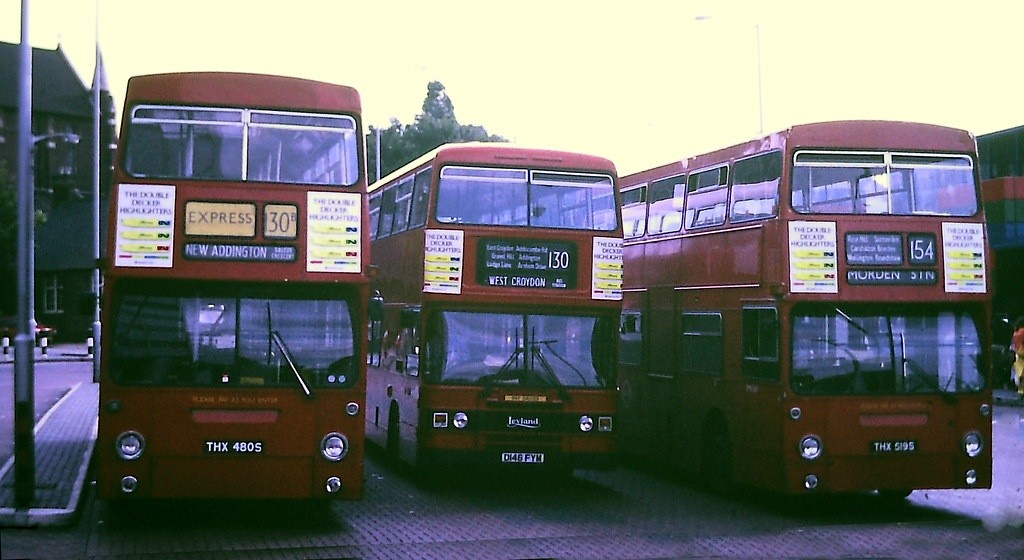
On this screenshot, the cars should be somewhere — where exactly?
[1,313,56,344]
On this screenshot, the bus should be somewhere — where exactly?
[94,52,380,510]
[314,144,639,471]
[482,120,1012,497]
